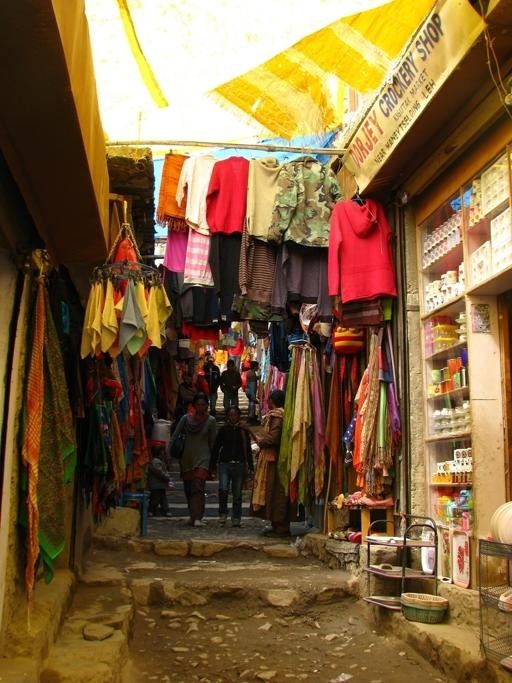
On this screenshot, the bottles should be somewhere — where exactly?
[467,179,484,227]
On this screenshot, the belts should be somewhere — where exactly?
[224,458,241,465]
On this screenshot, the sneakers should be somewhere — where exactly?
[264,530,291,537]
[327,527,386,544]
[193,515,241,527]
[147,511,172,517]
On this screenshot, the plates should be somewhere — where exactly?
[370,564,403,573]
[491,501,512,552]
[367,536,411,542]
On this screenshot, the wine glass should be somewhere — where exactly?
[421,210,461,267]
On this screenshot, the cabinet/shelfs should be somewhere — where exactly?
[363,519,438,616]
[416,144,509,588]
[477,537,512,671]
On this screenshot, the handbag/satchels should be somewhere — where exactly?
[332,324,364,355]
[343,301,385,328]
[170,432,186,459]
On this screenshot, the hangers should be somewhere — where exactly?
[348,187,367,208]
[299,145,307,156]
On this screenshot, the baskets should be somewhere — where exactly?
[400,592,449,624]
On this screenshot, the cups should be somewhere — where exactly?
[433,400,471,434]
[425,261,464,311]
[426,348,468,396]
[433,448,473,483]
[423,316,458,353]
[435,490,474,531]
[454,312,467,342]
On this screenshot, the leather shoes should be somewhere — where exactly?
[328,491,394,510]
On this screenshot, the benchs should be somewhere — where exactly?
[327,504,395,545]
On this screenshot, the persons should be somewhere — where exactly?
[172,352,264,425]
[172,394,218,526]
[212,405,253,527]
[146,448,172,519]
[251,390,292,538]
